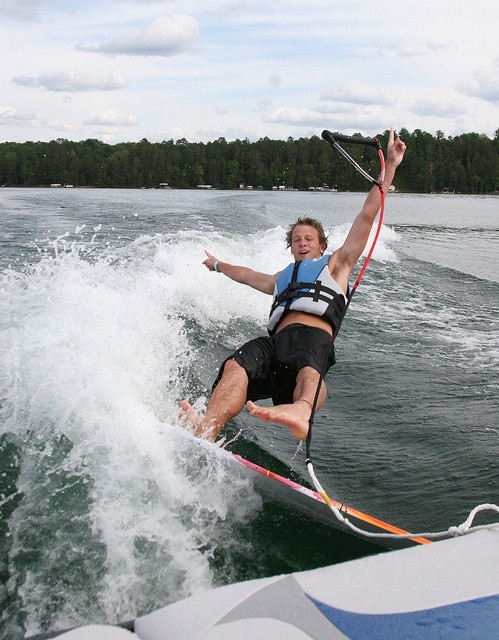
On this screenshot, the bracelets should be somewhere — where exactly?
[214,260,222,273]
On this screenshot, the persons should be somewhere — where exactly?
[178,126,408,445]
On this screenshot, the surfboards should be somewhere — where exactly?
[152,418,434,552]
